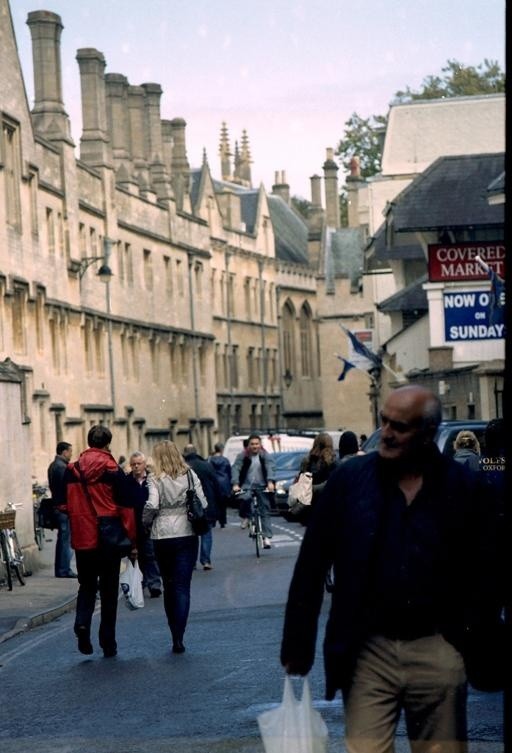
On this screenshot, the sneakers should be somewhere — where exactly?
[151,587,162,598]
[173,641,185,653]
[263,537,271,549]
[77,625,93,654]
[104,650,116,658]
[241,517,249,529]
[204,563,211,570]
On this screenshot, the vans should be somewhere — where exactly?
[223,431,343,466]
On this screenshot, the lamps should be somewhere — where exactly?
[68,253,114,285]
[282,368,292,387]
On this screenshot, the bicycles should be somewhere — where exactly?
[235,488,276,557]
[32,483,53,550]
[0,501,26,590]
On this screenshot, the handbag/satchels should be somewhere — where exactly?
[97,515,132,558]
[287,471,314,514]
[38,499,59,528]
[186,489,209,535]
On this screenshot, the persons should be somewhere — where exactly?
[46,442,77,578]
[312,431,367,494]
[62,425,208,657]
[359,434,367,446]
[280,384,504,753]
[184,434,277,570]
[298,433,343,593]
[453,431,481,472]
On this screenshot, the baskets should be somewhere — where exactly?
[0,511,15,529]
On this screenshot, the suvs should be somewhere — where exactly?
[360,419,488,457]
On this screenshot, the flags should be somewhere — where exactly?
[338,359,356,380]
[343,327,382,380]
[486,267,505,326]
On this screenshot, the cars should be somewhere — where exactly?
[267,450,340,512]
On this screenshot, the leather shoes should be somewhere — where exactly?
[66,573,77,578]
